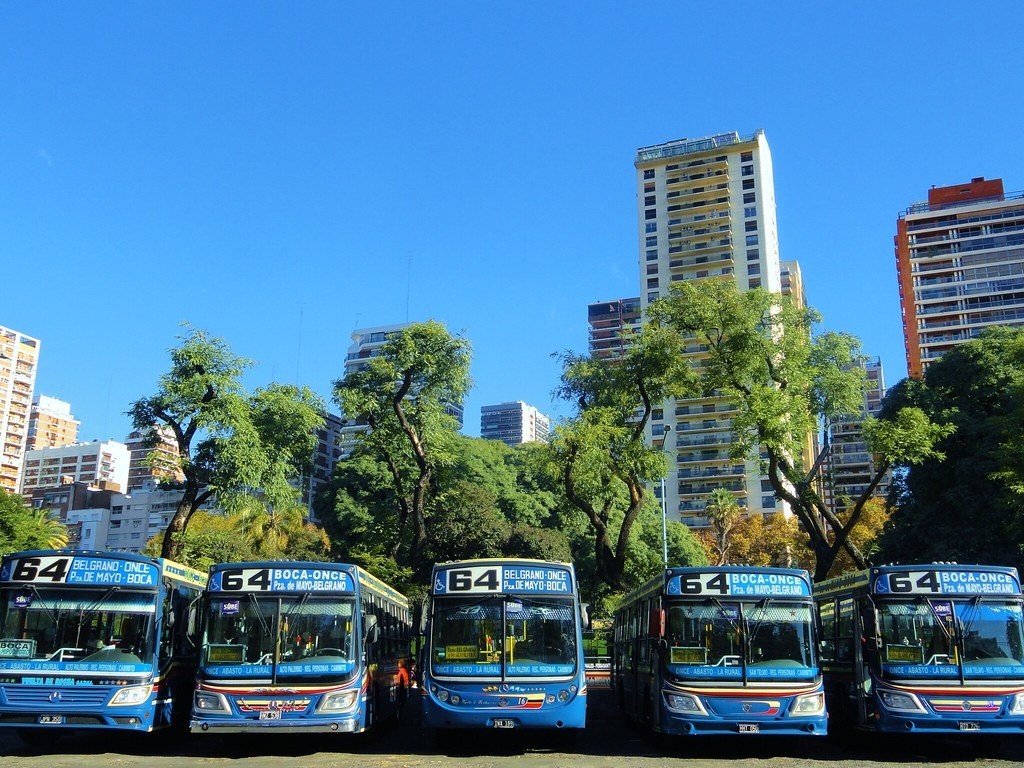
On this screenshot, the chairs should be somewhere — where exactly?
[313,632,332,655]
[534,629,560,658]
[122,618,134,637]
[35,616,106,658]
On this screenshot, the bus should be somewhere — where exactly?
[182,560,420,744]
[604,564,830,744]
[807,560,1024,741]
[411,558,588,738]
[0,549,208,754]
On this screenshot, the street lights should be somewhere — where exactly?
[658,424,670,570]
